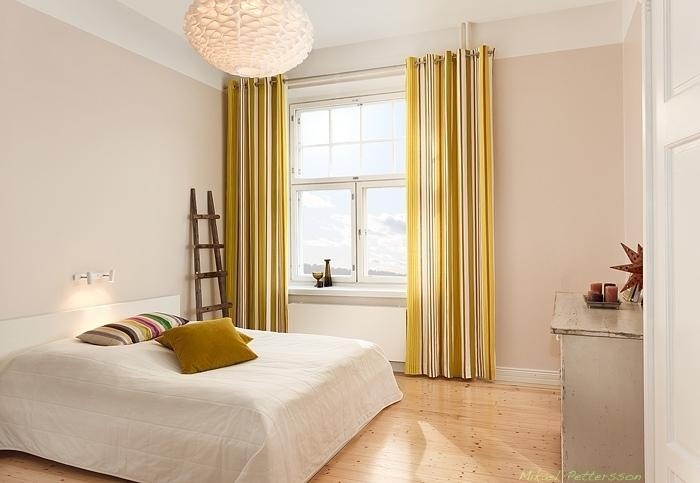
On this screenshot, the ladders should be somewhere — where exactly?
[192,188,233,321]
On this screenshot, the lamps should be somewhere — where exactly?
[75,272,94,284]
[97,271,114,282]
[182,0,313,78]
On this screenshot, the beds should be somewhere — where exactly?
[0,322,404,483]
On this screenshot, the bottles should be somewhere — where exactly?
[324,259,332,287]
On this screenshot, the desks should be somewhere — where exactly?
[552,291,645,482]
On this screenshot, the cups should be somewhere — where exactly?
[317,281,323,287]
[591,283,602,294]
[588,291,599,300]
[604,283,616,300]
[606,286,618,302]
[591,294,603,302]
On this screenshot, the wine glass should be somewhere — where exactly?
[312,273,324,287]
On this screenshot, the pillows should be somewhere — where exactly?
[76,311,188,346]
[162,317,258,374]
[155,332,253,351]
